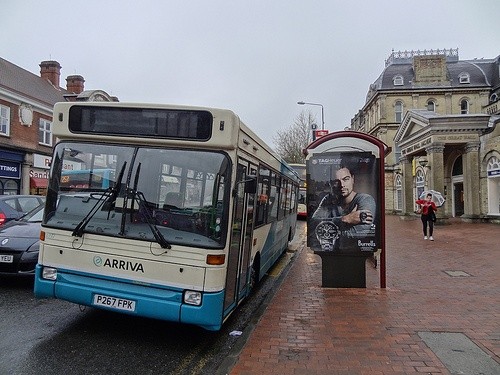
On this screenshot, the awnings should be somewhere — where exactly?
[30,176,48,189]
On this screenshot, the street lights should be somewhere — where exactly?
[296,100,325,132]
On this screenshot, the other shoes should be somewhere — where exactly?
[424,236,428,239]
[429,236,433,240]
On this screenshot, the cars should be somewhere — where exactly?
[0,194,46,228]
[0,192,111,290]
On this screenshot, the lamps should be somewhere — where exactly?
[419,150,431,169]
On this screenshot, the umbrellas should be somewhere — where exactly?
[419,190,446,208]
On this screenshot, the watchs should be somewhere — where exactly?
[360,210,367,224]
[315,220,342,251]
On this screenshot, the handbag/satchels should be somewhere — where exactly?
[432,210,437,223]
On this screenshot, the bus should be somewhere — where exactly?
[287,163,308,219]
[32,97,301,334]
[57,167,181,210]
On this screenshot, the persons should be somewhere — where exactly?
[308,164,375,239]
[415,194,437,241]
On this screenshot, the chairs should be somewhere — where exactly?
[163,192,183,209]
